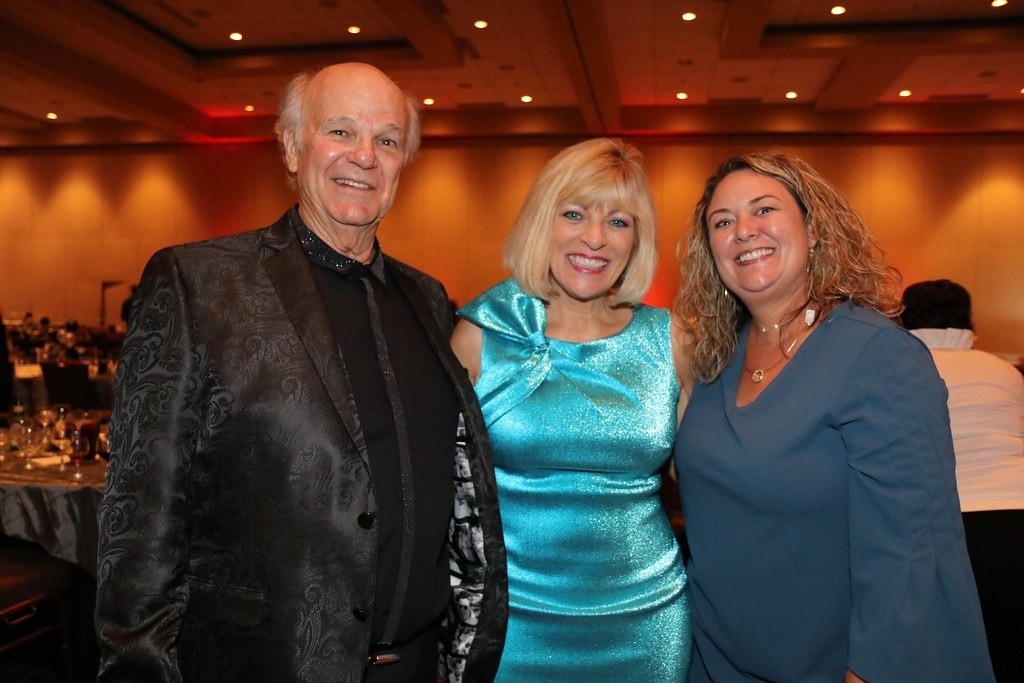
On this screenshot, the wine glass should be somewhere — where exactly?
[2,404,111,481]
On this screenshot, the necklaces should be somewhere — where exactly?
[744,326,809,383]
[754,300,813,334]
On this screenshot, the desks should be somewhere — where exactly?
[0,357,121,573]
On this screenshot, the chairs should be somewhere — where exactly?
[40,361,100,409]
[0,531,100,683]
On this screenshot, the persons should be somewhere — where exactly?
[9,313,124,408]
[450,136,696,683]
[673,149,994,683]
[0,320,15,427]
[93,61,509,683]
[894,279,1024,683]
